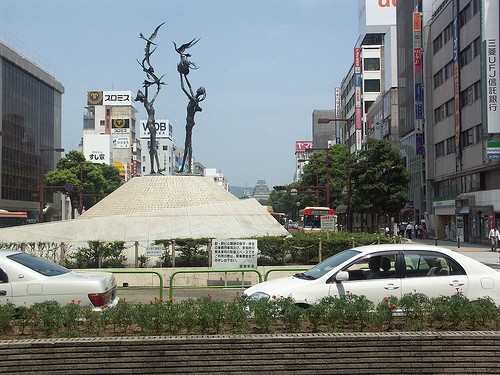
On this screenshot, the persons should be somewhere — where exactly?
[174,73,206,173]
[134,83,166,173]
[445,224,449,239]
[488,226,500,252]
[386,223,426,240]
[366,256,382,279]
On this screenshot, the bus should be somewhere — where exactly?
[299,206,335,231]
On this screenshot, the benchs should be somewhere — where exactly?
[426,257,463,276]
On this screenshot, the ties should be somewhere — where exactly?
[494,230,496,238]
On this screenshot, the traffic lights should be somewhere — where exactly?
[43,203,47,209]
[272,185,287,191]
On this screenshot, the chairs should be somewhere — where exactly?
[381,258,391,272]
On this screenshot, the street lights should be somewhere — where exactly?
[304,146,331,209]
[39,147,65,222]
[318,117,353,232]
[80,161,86,215]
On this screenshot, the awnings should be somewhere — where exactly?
[400,207,412,216]
[458,207,470,213]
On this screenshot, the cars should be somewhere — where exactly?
[286,219,298,229]
[242,243,500,320]
[0,248,121,321]
